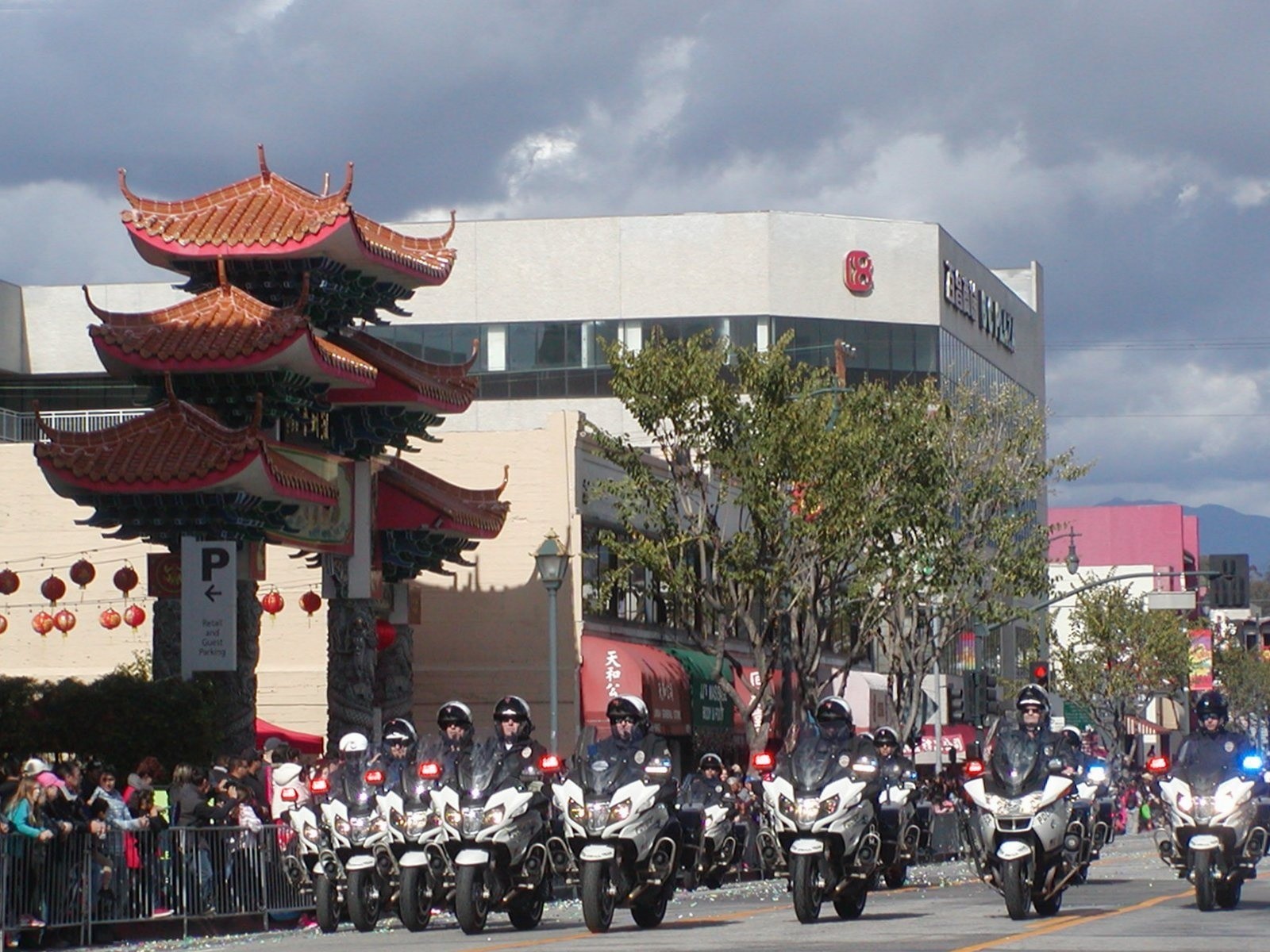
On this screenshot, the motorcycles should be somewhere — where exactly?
[283,732,1270,936]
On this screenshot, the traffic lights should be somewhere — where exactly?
[1031,663,1048,691]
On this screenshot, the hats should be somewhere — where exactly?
[37,771,65,788]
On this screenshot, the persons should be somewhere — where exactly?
[567,695,678,825]
[465,695,552,806]
[721,749,763,824]
[988,683,1077,795]
[1058,724,1111,797]
[0,750,339,950]
[676,753,735,819]
[426,699,484,792]
[1171,693,1269,791]
[1111,756,1169,835]
[325,732,371,813]
[916,764,974,820]
[367,716,420,803]
[777,694,880,813]
[873,727,920,818]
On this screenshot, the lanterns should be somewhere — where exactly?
[0,553,398,654]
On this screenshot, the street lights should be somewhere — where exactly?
[932,524,1083,776]
[530,529,575,751]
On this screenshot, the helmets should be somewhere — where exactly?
[337,682,1231,776]
